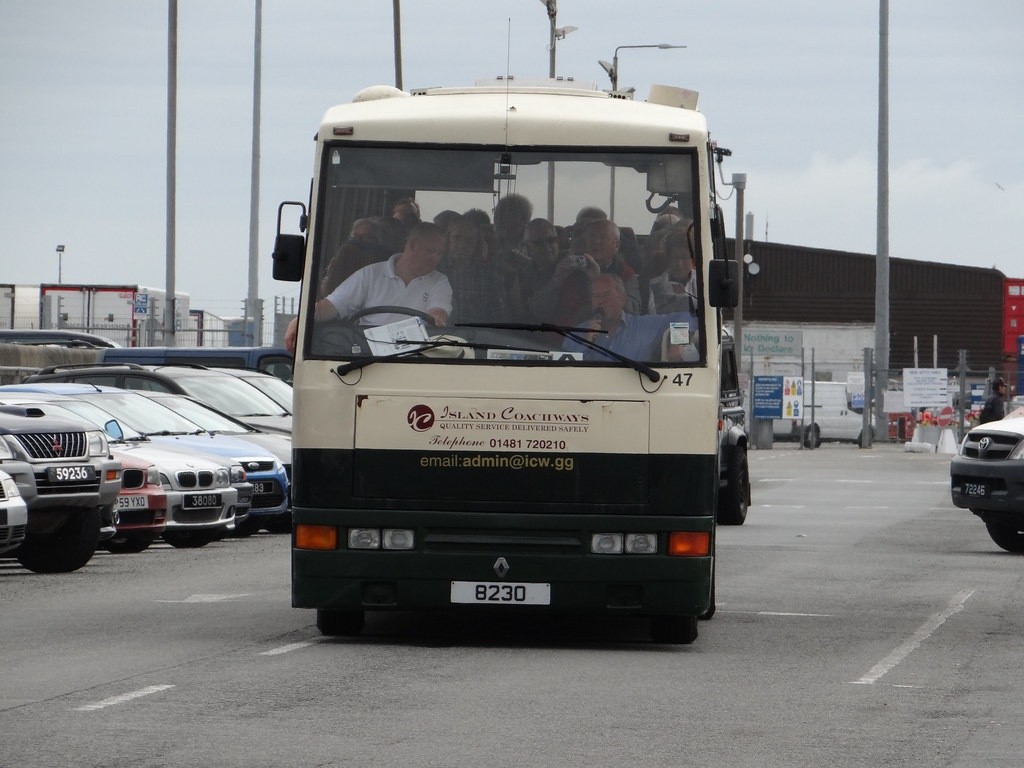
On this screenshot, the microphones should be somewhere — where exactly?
[592,307,604,337]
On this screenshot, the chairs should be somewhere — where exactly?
[325,239,389,296]
[619,226,638,275]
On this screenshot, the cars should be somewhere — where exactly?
[211,365,293,434]
[101,447,169,555]
[0,382,290,539]
[95,346,294,389]
[1,330,120,349]
[0,391,239,548]
[0,366,42,386]
[149,434,253,529]
[862,399,915,442]
[950,417,1024,553]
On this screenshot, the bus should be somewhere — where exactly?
[269,75,737,620]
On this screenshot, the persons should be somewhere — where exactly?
[562,274,698,363]
[317,194,699,322]
[284,222,453,353]
[979,378,1006,424]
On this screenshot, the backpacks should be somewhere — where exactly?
[979,397,998,424]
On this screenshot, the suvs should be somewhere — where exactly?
[9,339,98,351]
[773,381,876,449]
[0,400,124,573]
[717,327,752,525]
[18,361,293,486]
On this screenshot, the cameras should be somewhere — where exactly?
[570,255,587,268]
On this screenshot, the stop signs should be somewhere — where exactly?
[937,407,953,428]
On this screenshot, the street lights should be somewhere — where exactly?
[56,244,65,283]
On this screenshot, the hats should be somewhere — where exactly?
[993,377,1007,386]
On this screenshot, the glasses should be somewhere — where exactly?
[528,237,558,247]
[348,233,374,240]
